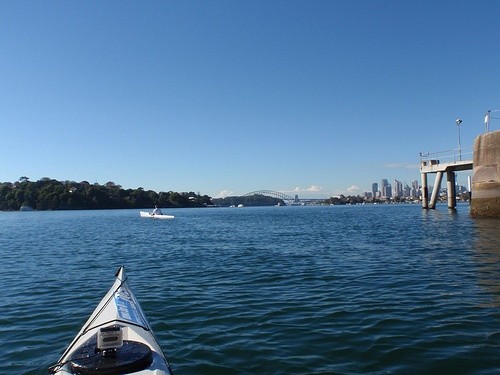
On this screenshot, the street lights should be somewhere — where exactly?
[456,119,463,162]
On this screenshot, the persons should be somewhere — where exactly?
[149,204,163,216]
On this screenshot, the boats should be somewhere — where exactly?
[47,264,177,375]
[238,204,244,207]
[140,211,174,220]
